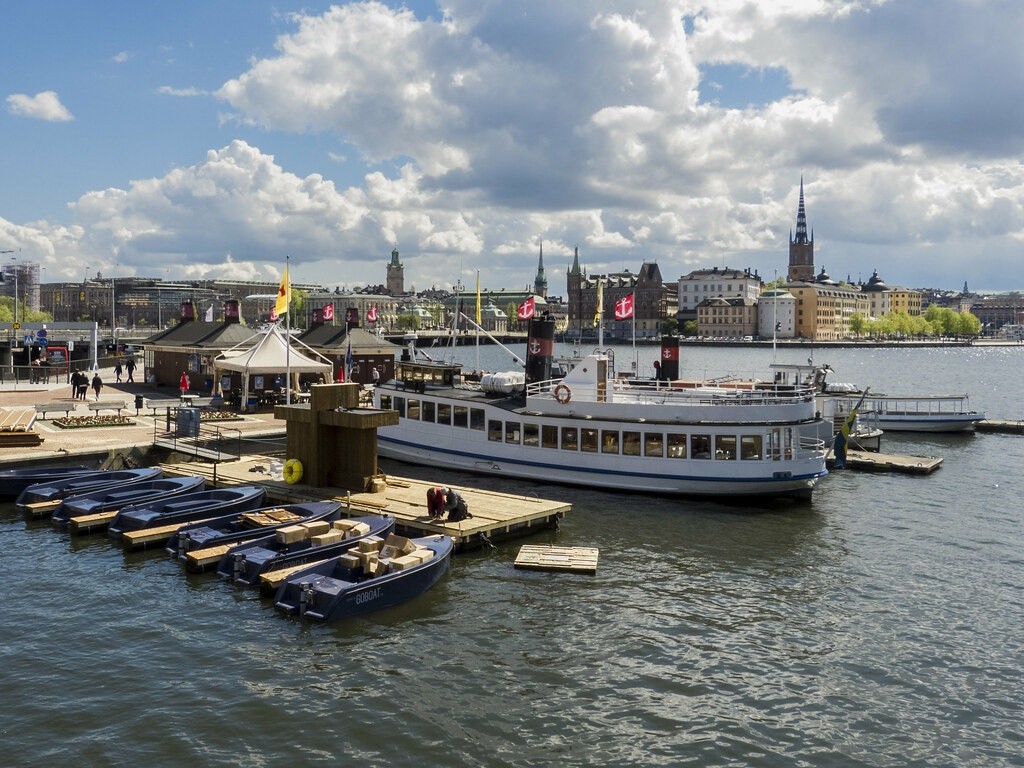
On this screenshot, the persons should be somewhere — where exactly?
[33,359,41,384]
[441,487,473,522]
[373,367,379,387]
[71,369,81,399]
[124,356,137,383]
[78,373,90,400]
[92,373,103,402]
[113,361,123,383]
[42,359,51,385]
[427,487,445,520]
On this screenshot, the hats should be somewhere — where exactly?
[440,486,449,495]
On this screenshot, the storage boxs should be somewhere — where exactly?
[276,520,434,578]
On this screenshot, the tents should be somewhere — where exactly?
[213,323,333,413]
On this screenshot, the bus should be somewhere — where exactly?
[47,347,68,375]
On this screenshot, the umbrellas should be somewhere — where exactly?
[337,367,344,384]
[180,373,189,403]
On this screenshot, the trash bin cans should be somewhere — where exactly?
[176,408,200,437]
[134,395,143,409]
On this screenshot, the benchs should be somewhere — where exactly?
[145,399,180,415]
[89,401,127,416]
[35,403,76,420]
[191,397,224,412]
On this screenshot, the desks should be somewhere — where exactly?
[237,390,312,398]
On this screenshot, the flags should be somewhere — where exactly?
[366,306,378,323]
[593,284,605,327]
[275,265,292,315]
[474,272,481,326]
[517,296,534,321]
[322,304,333,321]
[345,343,352,375]
[269,305,279,321]
[614,292,634,320]
[833,395,864,469]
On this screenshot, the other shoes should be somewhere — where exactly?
[467,513,473,518]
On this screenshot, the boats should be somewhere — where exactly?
[273,534,456,622]
[106,486,269,552]
[373,278,986,497]
[0,464,163,521]
[217,513,396,586]
[163,501,341,572]
[49,475,206,534]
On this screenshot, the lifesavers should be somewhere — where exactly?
[282,458,304,485]
[554,384,572,404]
